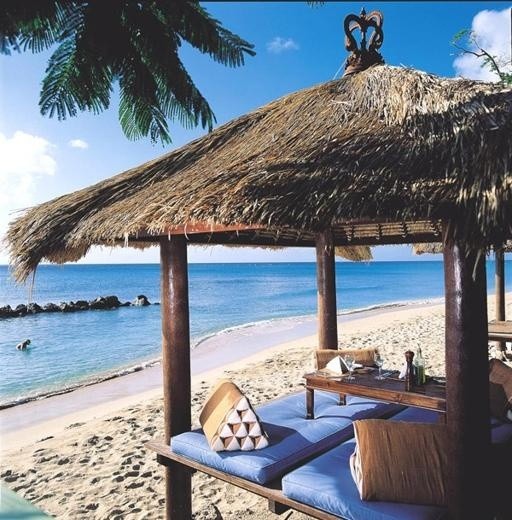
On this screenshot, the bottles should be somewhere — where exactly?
[405,345,426,390]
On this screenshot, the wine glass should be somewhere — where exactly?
[345,349,387,382]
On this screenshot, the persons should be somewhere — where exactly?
[16,339,31,351]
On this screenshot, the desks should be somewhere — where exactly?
[303,368,446,426]
